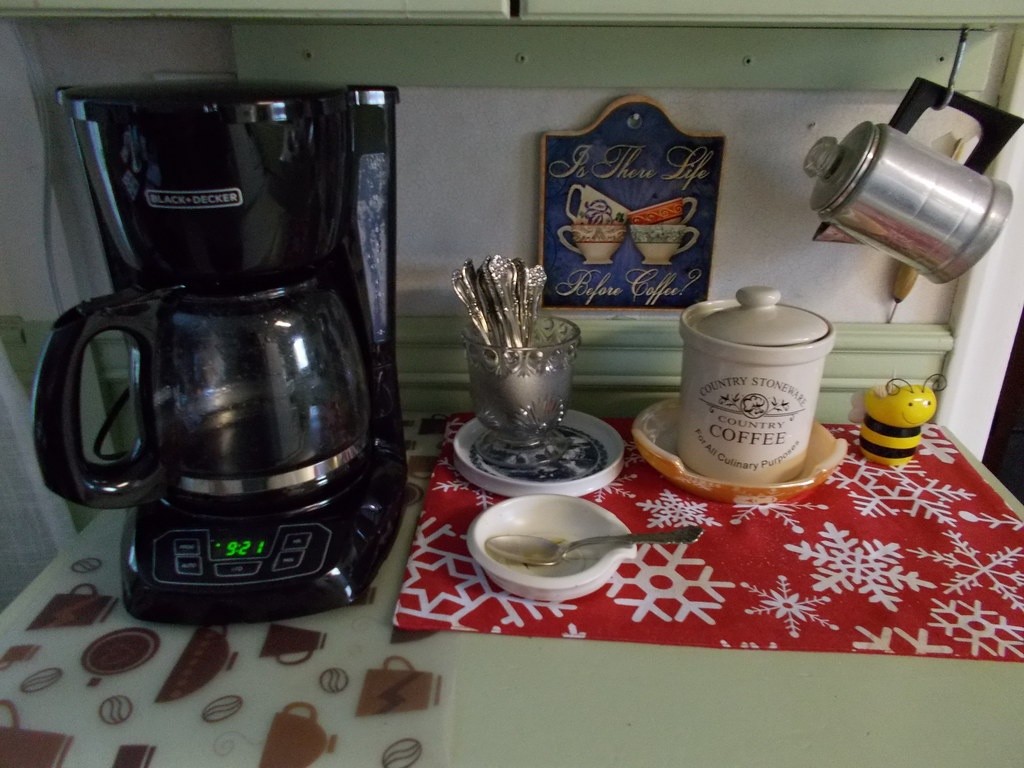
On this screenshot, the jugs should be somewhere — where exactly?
[801,76,1024,285]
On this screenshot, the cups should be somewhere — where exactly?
[676,285,837,486]
[627,196,698,224]
[565,183,630,225]
[556,225,627,265]
[629,224,700,266]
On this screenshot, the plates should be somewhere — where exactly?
[452,409,625,498]
[631,397,849,504]
[465,492,638,603]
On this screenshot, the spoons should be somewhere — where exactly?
[485,525,705,567]
[451,252,548,422]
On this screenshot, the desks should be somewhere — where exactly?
[0,416,1024,766]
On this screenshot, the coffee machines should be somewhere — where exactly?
[32,73,406,630]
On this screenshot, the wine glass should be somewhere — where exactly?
[459,315,583,469]
